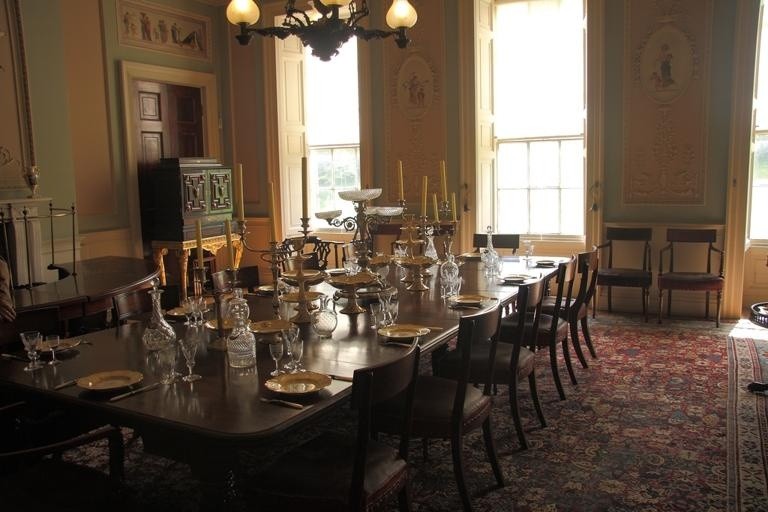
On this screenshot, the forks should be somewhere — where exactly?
[377,340,411,347]
[448,306,480,310]
[260,396,303,409]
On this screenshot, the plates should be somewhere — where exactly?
[369,254,394,267]
[377,324,431,339]
[535,260,555,265]
[249,319,295,332]
[258,269,347,301]
[41,337,81,354]
[167,307,185,318]
[447,294,491,306]
[263,370,331,397]
[75,368,144,391]
[204,319,251,330]
[462,252,484,261]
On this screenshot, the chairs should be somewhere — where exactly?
[593,227,653,322]
[656,228,725,325]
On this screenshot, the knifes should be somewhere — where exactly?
[110,382,159,402]
[326,372,354,383]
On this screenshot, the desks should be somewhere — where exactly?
[151,234,243,300]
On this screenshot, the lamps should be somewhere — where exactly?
[224,1,419,61]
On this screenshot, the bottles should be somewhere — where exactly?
[441,254,458,279]
[481,226,499,268]
[220,288,256,368]
[228,370,260,418]
[344,251,362,277]
[145,343,176,386]
[142,287,178,350]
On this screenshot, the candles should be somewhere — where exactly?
[194,155,459,271]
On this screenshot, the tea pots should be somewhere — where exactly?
[308,295,339,339]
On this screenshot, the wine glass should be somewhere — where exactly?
[19,329,63,371]
[182,296,206,328]
[279,269,326,326]
[370,290,399,330]
[185,381,204,420]
[271,324,304,376]
[178,337,204,381]
[439,279,461,300]
[322,273,378,316]
[396,255,437,292]
